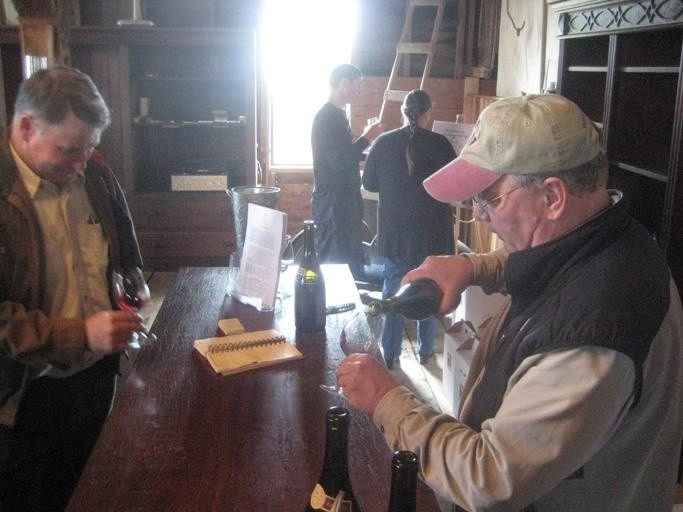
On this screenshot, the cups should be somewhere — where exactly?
[224,251,241,298]
[232,185,281,260]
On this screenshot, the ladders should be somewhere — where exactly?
[358,0,447,201]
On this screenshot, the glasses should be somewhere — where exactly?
[470,182,534,209]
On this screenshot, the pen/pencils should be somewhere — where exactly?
[326,303,355,314]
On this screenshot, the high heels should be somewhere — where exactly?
[386,354,401,369]
[419,350,438,367]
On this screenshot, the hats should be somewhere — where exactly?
[423,93,603,205]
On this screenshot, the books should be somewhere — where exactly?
[191,329,304,377]
[236,202,288,310]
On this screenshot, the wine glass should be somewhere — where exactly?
[320,310,386,403]
[109,265,157,349]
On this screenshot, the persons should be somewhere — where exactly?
[336,93,681,512]
[311,64,382,280]
[362,89,458,368]
[2,67,151,512]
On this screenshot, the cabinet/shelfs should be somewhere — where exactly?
[69,27,257,271]
[543,0,682,309]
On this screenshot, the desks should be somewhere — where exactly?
[67,263,438,508]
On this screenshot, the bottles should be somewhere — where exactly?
[305,405,419,512]
[370,278,462,321]
[294,219,326,331]
